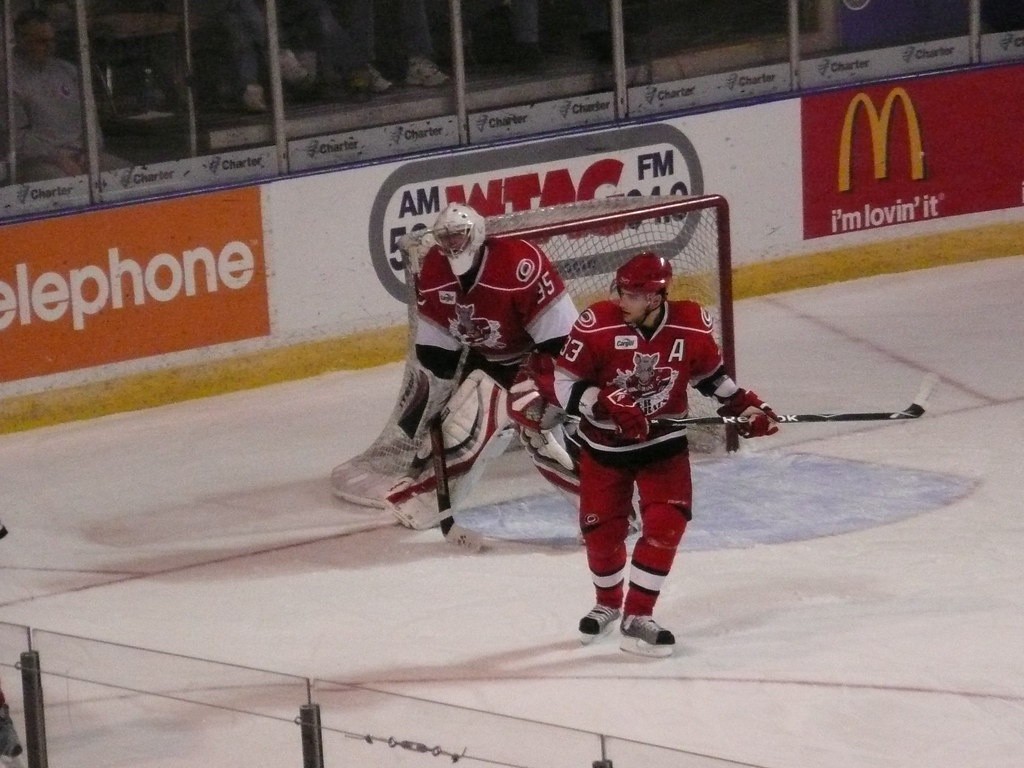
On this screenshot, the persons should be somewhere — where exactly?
[307,0,631,96]
[387,202,642,544]
[553,252,779,658]
[164,0,311,112]
[0,11,134,182]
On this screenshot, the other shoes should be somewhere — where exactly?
[338,63,372,92]
[513,42,546,73]
[295,77,337,104]
[581,31,612,63]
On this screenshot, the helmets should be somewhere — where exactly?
[617,252,673,295]
[433,204,487,276]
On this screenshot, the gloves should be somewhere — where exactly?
[718,391,779,439]
[593,384,650,442]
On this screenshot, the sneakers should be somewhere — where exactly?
[576,603,620,646]
[240,82,268,114]
[270,47,306,81]
[619,614,676,658]
[362,63,393,95]
[406,56,449,88]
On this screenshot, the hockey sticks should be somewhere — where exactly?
[647,370,938,430]
[428,408,583,554]
